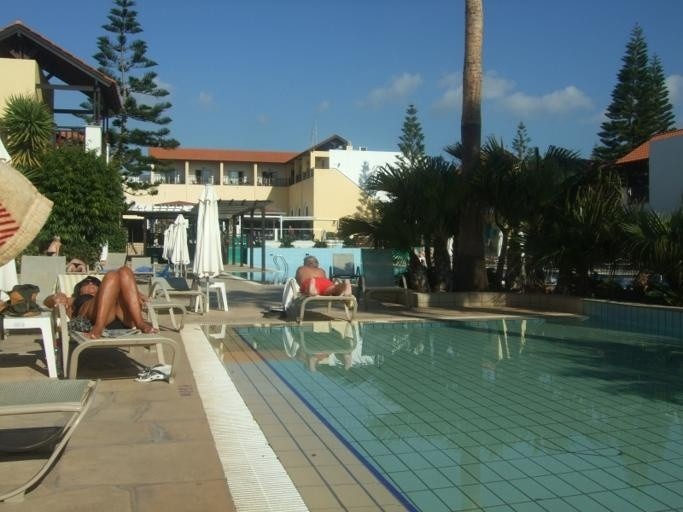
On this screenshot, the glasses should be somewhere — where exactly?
[81,279,99,287]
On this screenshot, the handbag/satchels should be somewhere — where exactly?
[6,284,40,312]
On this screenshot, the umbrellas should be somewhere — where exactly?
[162,183,224,312]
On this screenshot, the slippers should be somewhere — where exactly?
[135,363,171,382]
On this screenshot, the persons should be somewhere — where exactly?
[296,256,353,296]
[43,265,159,340]
[296,350,353,372]
[46,235,62,256]
[67,258,86,273]
